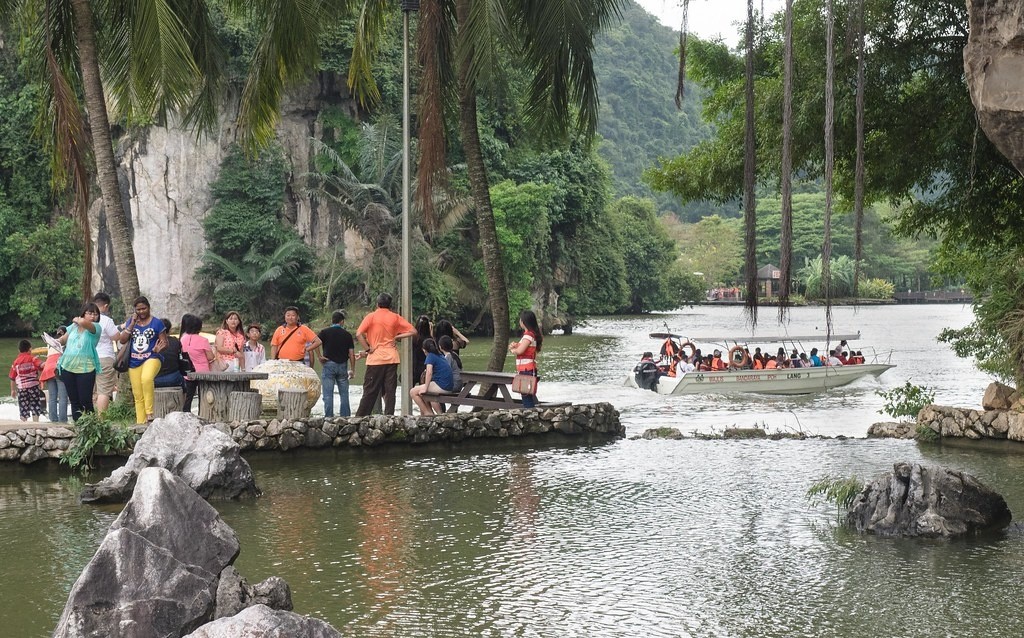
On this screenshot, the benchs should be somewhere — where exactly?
[514,401,572,409]
[420,392,471,404]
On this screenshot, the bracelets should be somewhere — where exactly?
[242,369,245,370]
[124,326,132,334]
[365,349,371,352]
[305,348,307,352]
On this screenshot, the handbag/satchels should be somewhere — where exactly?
[113,336,132,371]
[512,374,537,395]
[179,358,190,376]
[182,352,195,373]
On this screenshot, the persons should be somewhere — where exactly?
[642,352,665,371]
[353,292,418,417]
[8,340,45,424]
[412,315,433,388]
[119,296,186,424]
[508,311,543,408]
[40,291,125,423]
[410,338,454,415]
[428,334,463,414]
[271,305,323,369]
[745,346,843,369]
[312,311,356,418]
[240,324,267,373]
[668,348,728,380]
[178,312,216,416]
[214,310,246,373]
[429,320,470,355]
[834,338,865,365]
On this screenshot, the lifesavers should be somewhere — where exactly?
[680,342,695,358]
[729,345,747,367]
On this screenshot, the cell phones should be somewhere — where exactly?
[134,307,136,322]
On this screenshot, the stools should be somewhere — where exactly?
[227,391,263,423]
[155,386,184,411]
[277,388,309,421]
[154,389,181,419]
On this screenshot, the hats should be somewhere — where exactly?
[715,350,722,356]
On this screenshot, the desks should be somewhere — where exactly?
[447,369,541,414]
[187,371,268,422]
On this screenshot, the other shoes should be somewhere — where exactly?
[147,414,155,422]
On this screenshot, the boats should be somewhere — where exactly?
[621,364,898,396]
